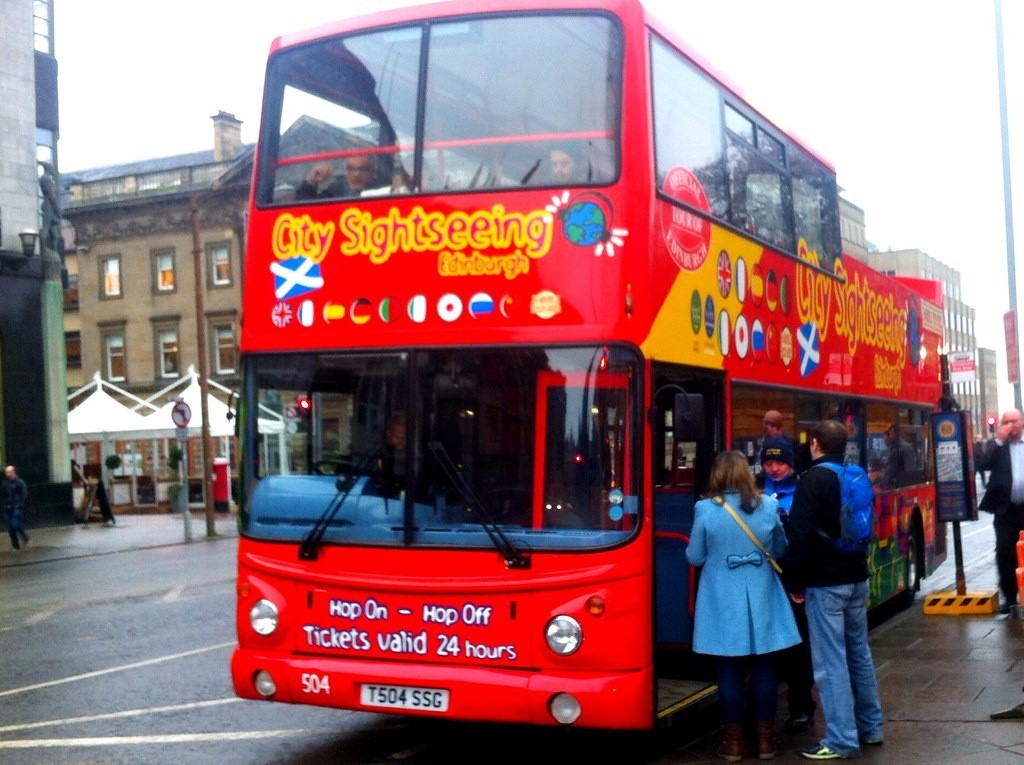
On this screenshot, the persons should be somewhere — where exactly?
[0,465,30,551]
[361,410,886,761]
[866,425,927,494]
[300,145,611,199]
[974,409,1024,613]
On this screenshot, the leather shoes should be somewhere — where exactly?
[784,713,815,729]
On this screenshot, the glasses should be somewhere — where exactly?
[346,163,368,171]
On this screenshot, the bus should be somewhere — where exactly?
[226,0,943,729]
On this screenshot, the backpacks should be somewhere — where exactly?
[800,462,877,552]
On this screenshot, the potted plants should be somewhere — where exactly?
[167,446,185,512]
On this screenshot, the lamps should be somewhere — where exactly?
[19,225,38,256]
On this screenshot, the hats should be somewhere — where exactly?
[548,140,583,164]
[760,437,798,467]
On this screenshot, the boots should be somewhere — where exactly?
[717,721,744,761]
[756,720,780,759]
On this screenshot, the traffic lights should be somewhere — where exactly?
[988,418,995,433]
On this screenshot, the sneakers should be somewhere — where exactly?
[802,743,846,759]
[862,738,884,745]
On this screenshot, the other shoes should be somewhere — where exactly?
[1001,602,1019,614]
